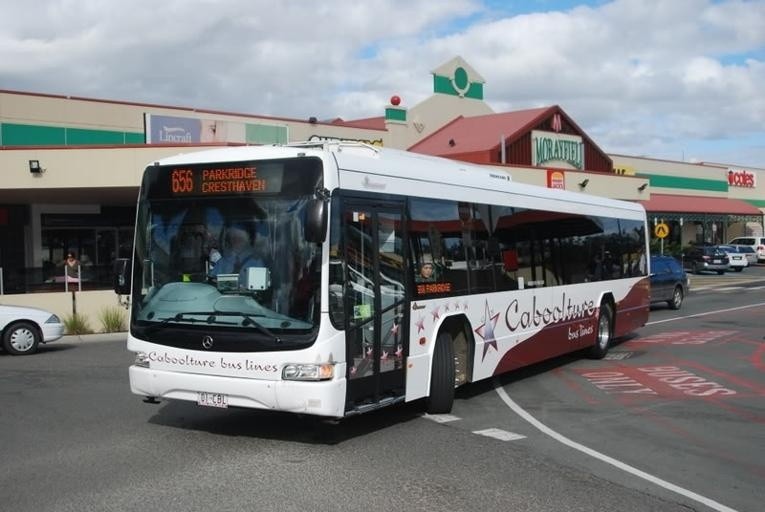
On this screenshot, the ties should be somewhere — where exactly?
[233,256,240,274]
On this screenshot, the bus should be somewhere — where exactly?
[116,134,651,425]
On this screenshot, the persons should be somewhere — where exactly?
[61,250,83,291]
[414,262,436,283]
[208,229,266,286]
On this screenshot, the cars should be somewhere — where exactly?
[677,244,758,275]
[1,304,65,355]
[650,256,689,310]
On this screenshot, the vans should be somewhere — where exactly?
[730,237,765,262]
